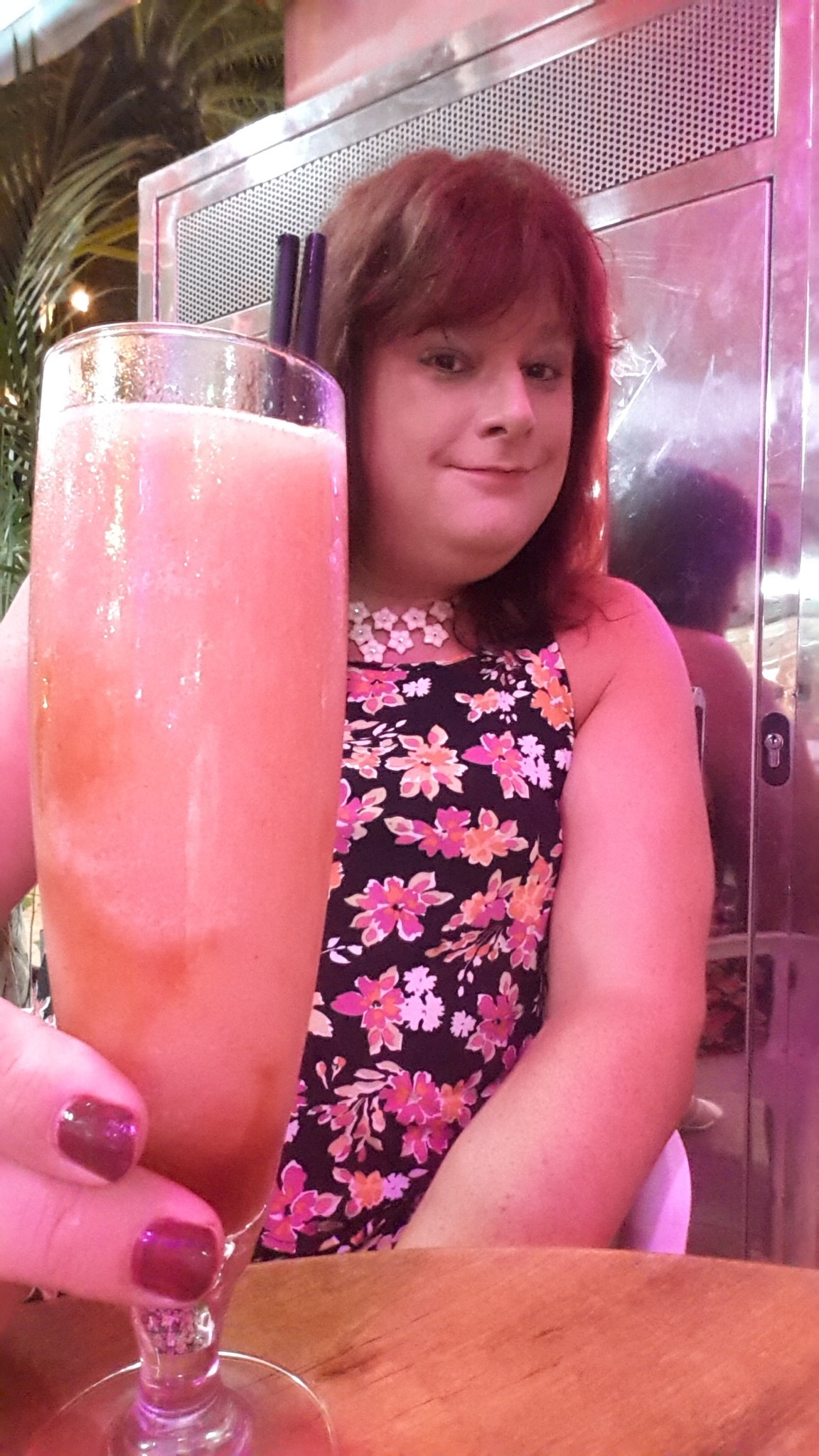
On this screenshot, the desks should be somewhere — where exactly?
[0,1247,819,1456]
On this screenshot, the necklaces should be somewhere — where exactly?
[346,598,455,667]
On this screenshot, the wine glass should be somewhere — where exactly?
[31,319,348,1456]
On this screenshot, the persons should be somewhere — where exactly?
[2,146,718,1308]
[605,464,817,1055]
